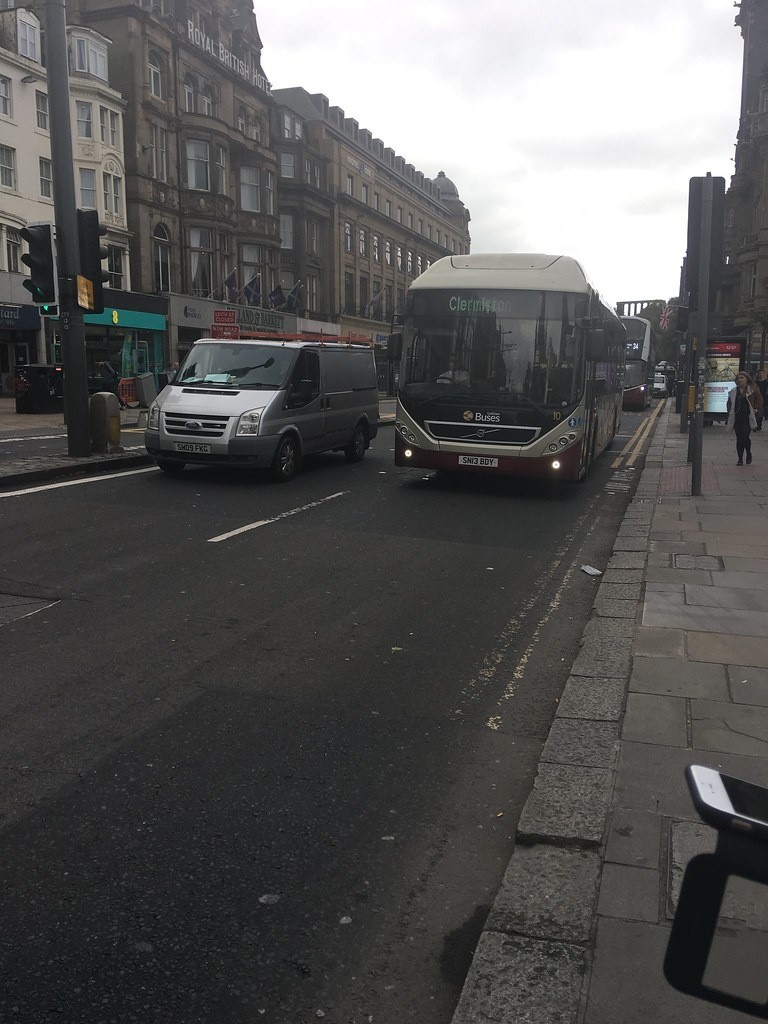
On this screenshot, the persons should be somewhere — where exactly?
[96,359,127,410]
[725,371,763,466]
[170,361,180,371]
[752,368,768,433]
[437,354,471,388]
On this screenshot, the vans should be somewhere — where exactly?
[144,329,380,481]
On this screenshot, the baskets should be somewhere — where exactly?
[711,360,717,368]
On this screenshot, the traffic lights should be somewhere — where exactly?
[77,208,110,314]
[19,221,60,317]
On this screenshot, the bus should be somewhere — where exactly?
[617,316,657,407]
[387,254,628,489]
[655,365,677,396]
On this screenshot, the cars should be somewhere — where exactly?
[653,375,668,398]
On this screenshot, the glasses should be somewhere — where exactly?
[449,359,459,363]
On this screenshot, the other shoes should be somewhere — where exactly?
[736,460,743,466]
[746,453,752,464]
[752,425,761,432]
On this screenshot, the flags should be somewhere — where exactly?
[224,269,236,292]
[243,273,261,307]
[267,285,286,311]
[288,284,303,313]
[658,306,673,330]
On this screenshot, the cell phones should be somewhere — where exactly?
[685,764,768,839]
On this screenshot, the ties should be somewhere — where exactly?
[450,371,456,384]
